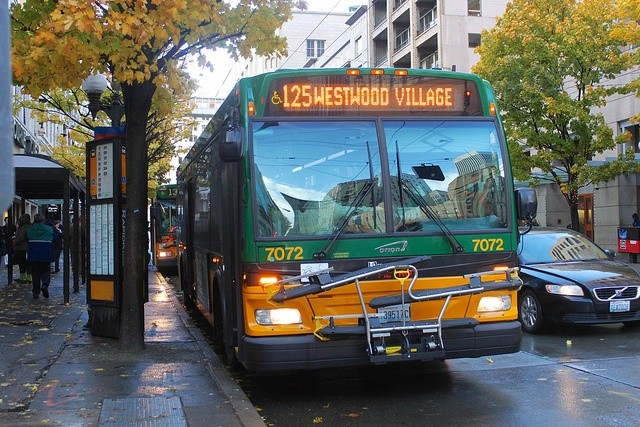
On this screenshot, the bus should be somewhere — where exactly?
[176,68,537,375]
[150,184,178,268]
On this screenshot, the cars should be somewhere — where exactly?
[516,225,640,333]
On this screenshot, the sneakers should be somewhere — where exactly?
[42,286,48,297]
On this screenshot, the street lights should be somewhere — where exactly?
[82,67,126,134]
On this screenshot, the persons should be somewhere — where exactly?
[12,213,33,283]
[45,212,60,272]
[53,218,64,249]
[26,212,54,298]
[631,212,640,262]
[2,216,16,269]
[68,214,86,275]
[0,225,8,262]
